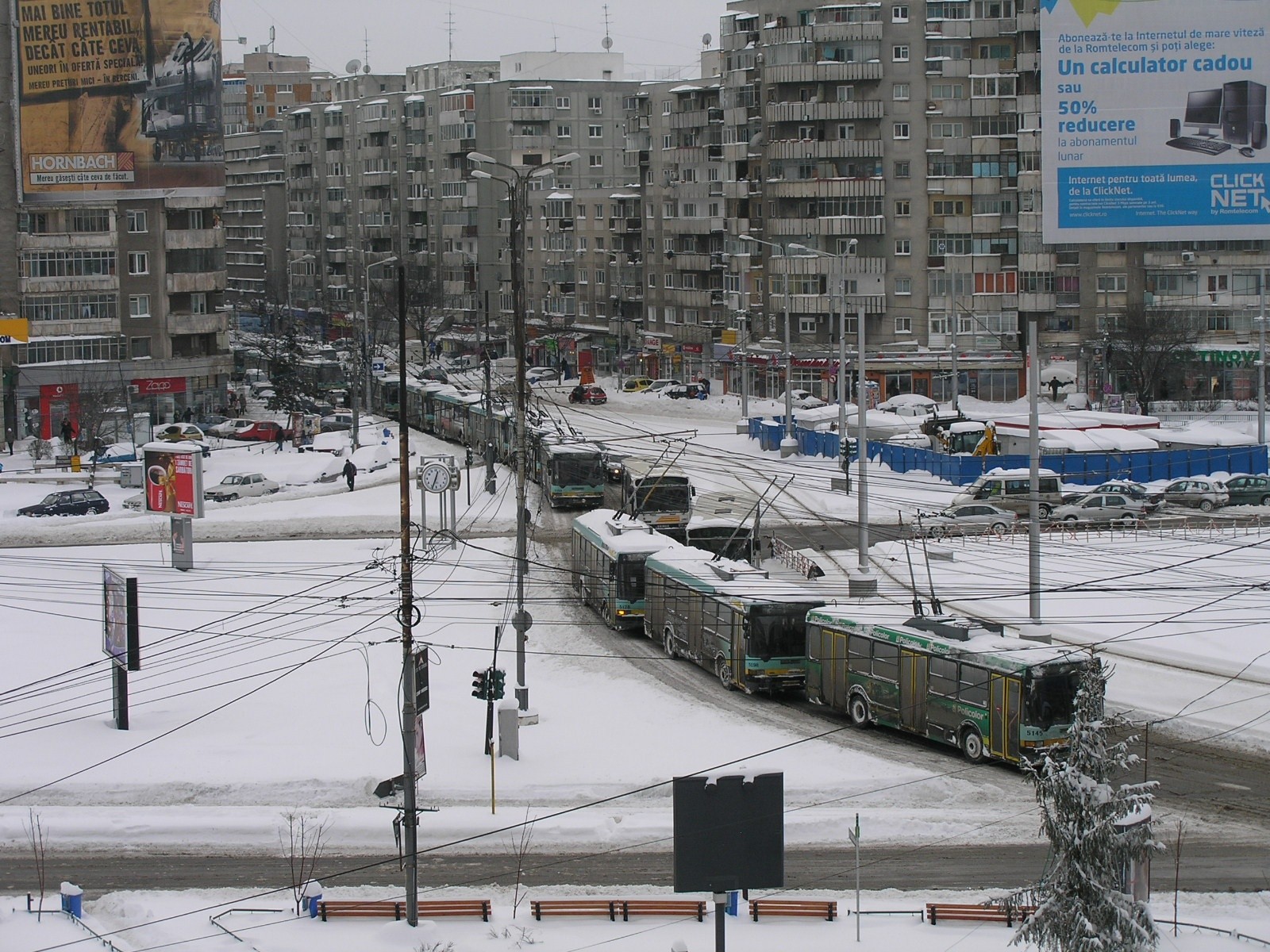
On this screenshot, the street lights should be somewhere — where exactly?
[289,253,317,323]
[363,255,398,412]
[739,233,851,471]
[469,147,583,718]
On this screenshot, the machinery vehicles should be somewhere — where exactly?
[938,421,999,456]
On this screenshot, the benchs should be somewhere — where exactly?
[530,901,619,921]
[749,900,837,921]
[618,900,707,922]
[316,900,491,922]
[925,902,1041,929]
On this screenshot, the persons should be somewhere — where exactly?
[890,384,900,398]
[173,407,195,424]
[275,427,287,454]
[1182,385,1188,401]
[526,354,533,365]
[60,417,76,444]
[429,341,442,360]
[219,392,247,419]
[701,379,710,395]
[172,531,184,554]
[1048,376,1063,402]
[561,357,568,371]
[6,428,15,455]
[342,459,357,492]
[1160,377,1168,400]
[1213,382,1222,400]
[415,720,425,765]
[574,384,586,403]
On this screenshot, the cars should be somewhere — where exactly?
[230,330,353,354]
[406,343,940,447]
[601,452,630,481]
[17,366,369,518]
[911,467,1270,539]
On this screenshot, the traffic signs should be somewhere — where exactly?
[372,359,384,375]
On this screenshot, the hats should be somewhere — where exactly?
[346,459,349,462]
[8,428,11,432]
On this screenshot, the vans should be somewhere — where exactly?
[951,471,1062,517]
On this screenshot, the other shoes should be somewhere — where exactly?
[275,450,277,454]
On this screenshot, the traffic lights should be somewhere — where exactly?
[466,446,473,464]
[471,669,487,700]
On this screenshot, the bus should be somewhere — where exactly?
[806,607,1106,766]
[685,491,761,566]
[571,508,684,631]
[644,547,825,694]
[619,457,697,535]
[230,349,604,511]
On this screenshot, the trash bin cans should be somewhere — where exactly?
[726,891,738,917]
[301,892,322,920]
[59,892,82,920]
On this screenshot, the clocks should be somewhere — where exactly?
[421,465,450,493]
